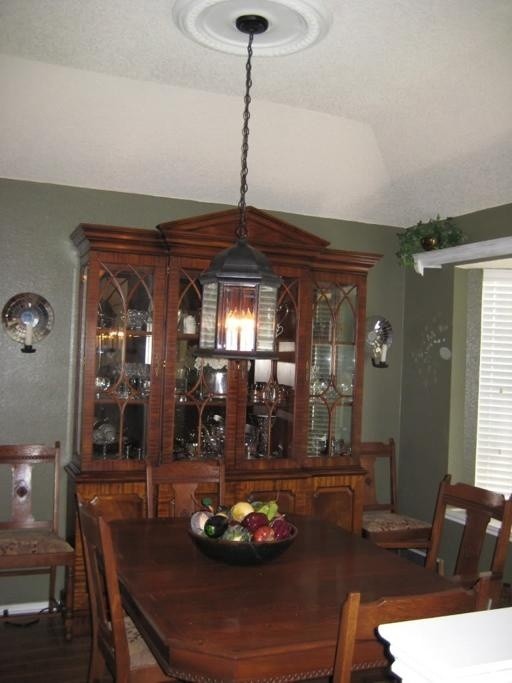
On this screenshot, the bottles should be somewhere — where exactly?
[176,364,190,401]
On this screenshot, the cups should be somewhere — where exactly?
[201,424,225,453]
[175,429,187,450]
[245,423,257,449]
[124,442,133,458]
[187,431,199,454]
[120,309,137,328]
[251,383,290,403]
[98,444,111,459]
[133,310,148,329]
[256,414,276,454]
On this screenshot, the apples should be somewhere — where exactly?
[242,512,267,531]
[269,517,290,540]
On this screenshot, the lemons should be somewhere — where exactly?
[230,502,252,520]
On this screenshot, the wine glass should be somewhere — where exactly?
[140,376,152,397]
[95,376,113,398]
[134,363,150,396]
[117,361,137,397]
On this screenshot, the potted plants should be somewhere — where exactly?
[396,213,470,268]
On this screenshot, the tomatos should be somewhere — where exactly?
[253,526,274,542]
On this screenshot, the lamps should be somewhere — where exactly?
[194,31,283,361]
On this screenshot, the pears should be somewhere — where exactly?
[258,501,278,522]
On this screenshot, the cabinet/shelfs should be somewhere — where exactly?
[63,205,384,618]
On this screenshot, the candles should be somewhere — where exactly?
[25,321,33,345]
[380,343,388,361]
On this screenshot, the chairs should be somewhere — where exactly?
[0,440,75,641]
[361,439,433,542]
[330,580,497,683]
[78,503,175,683]
[377,474,512,583]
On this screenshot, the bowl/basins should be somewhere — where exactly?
[204,369,245,399]
[314,437,343,455]
[187,518,298,565]
